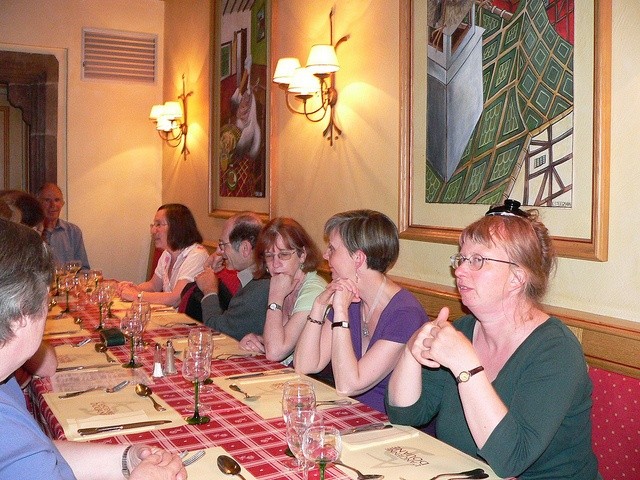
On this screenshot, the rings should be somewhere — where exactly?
[250,345,257,350]
[245,339,251,345]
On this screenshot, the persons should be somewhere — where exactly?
[37,184,90,279]
[1,190,45,234]
[291,210,435,440]
[0,219,189,479]
[383,212,602,479]
[14,342,59,392]
[239,217,335,385]
[194,211,269,342]
[115,203,212,308]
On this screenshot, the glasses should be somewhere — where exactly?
[217,239,241,252]
[150,220,170,229]
[262,249,299,262]
[448,253,522,271]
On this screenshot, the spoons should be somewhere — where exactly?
[229,384,262,401]
[217,353,252,360]
[94,342,115,363]
[334,459,384,480]
[134,383,167,412]
[217,454,247,480]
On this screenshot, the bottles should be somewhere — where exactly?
[166,340,178,375]
[152,342,164,379]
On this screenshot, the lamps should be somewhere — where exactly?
[157,115,174,134]
[272,57,302,89]
[306,44,341,82]
[161,101,182,121]
[287,67,320,101]
[149,104,165,123]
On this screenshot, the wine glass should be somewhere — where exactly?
[286,411,325,477]
[283,380,317,457]
[182,328,213,396]
[131,300,152,350]
[301,426,342,479]
[119,312,146,369]
[221,154,228,191]
[181,346,212,425]
[45,256,120,330]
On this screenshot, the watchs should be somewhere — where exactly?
[331,321,351,329]
[137,291,142,303]
[455,365,484,384]
[267,303,282,311]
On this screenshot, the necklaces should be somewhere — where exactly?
[361,278,386,337]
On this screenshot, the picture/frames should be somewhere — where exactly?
[208,0,277,225]
[397,1,610,263]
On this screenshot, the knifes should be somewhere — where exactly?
[77,420,173,435]
[340,422,384,437]
[225,369,296,380]
[56,363,120,373]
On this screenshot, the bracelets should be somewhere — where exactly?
[307,315,325,325]
[122,444,134,479]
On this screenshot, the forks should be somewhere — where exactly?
[51,338,92,349]
[58,380,130,398]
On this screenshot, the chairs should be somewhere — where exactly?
[576,367,637,480]
